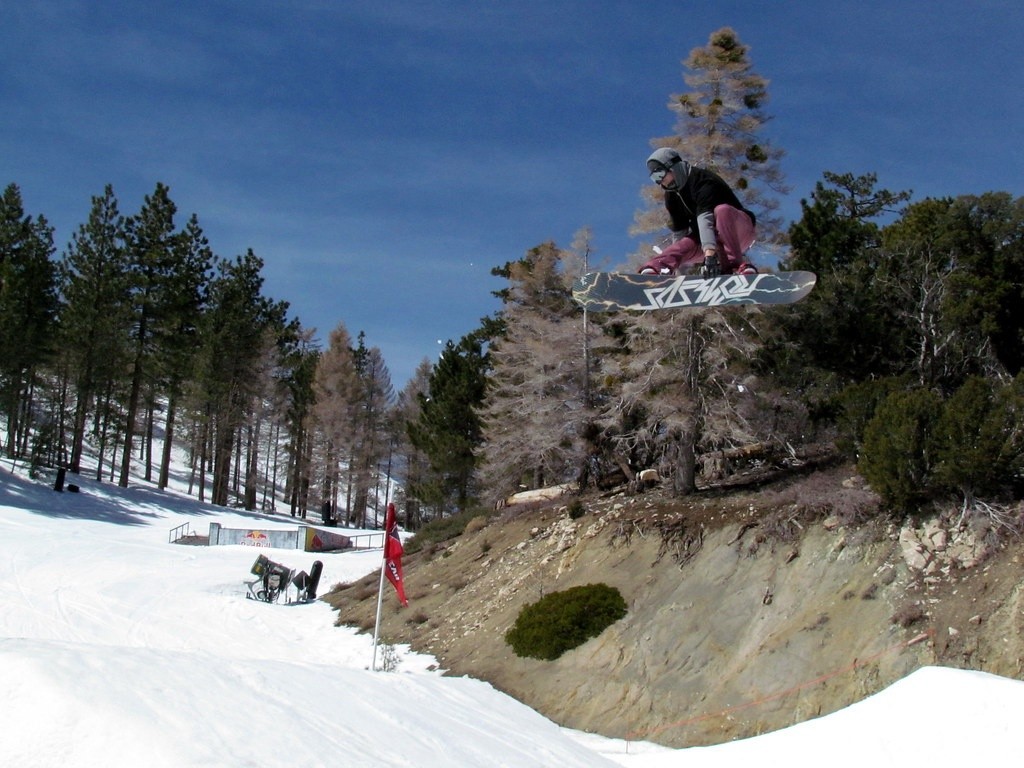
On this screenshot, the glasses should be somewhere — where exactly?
[648,167,667,185]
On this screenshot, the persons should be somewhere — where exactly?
[638,148,759,280]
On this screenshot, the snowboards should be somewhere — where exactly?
[572,270,816,313]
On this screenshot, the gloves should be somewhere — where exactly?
[701,252,721,281]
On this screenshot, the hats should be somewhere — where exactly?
[647,159,663,171]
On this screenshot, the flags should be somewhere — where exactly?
[384,505,407,605]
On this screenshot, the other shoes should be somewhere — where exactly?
[640,267,657,274]
[737,262,759,274]
